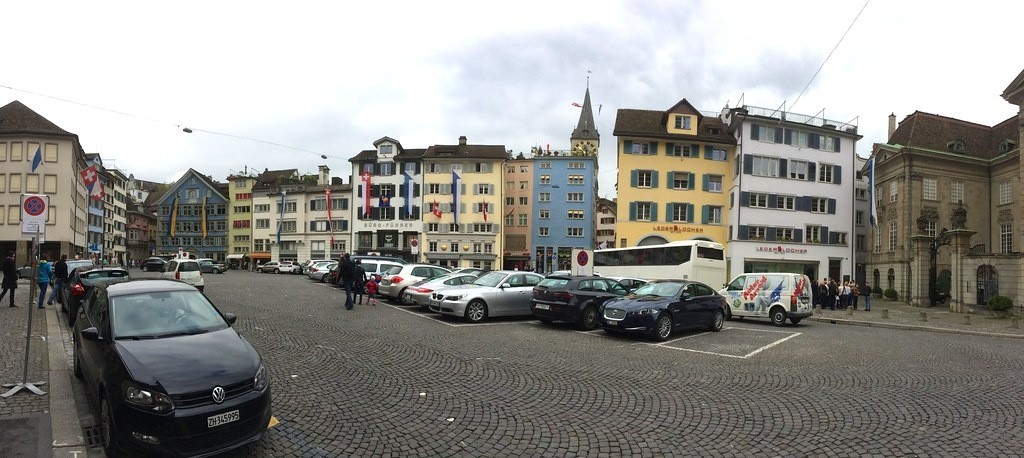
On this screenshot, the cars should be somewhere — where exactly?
[52,260,96,285]
[158,258,204,291]
[597,278,728,341]
[403,267,544,323]
[604,277,647,292]
[73,277,271,458]
[58,266,133,327]
[16,262,55,279]
[530,273,632,331]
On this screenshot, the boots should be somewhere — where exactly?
[373,298,376,305]
[366,296,370,305]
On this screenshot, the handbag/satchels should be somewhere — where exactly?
[836,296,840,300]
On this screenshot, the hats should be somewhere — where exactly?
[371,276,376,280]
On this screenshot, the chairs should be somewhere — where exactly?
[595,283,602,289]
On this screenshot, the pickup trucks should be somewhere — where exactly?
[197,259,226,274]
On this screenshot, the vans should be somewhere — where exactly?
[717,273,814,327]
[349,256,408,295]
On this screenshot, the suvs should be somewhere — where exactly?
[140,260,167,272]
[256,261,280,274]
[379,264,453,305]
[278,261,302,275]
[304,259,339,283]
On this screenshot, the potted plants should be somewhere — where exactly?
[884,289,897,301]
[987,295,1013,319]
[873,287,882,298]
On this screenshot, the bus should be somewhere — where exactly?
[592,240,728,293]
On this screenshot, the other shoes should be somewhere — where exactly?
[868,309,870,311]
[354,301,356,303]
[38,305,45,309]
[10,304,18,307]
[345,303,348,306]
[359,302,362,304]
[47,301,53,305]
[863,309,868,311]
[347,306,352,310]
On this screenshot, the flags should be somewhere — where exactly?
[80,164,105,200]
[31,146,41,172]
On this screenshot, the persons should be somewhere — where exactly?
[812,278,858,311]
[353,259,367,305]
[38,254,52,309]
[47,255,69,304]
[92,255,96,262]
[864,283,872,312]
[0,250,19,307]
[337,253,355,310]
[129,259,142,268]
[366,276,379,306]
[75,253,81,260]
